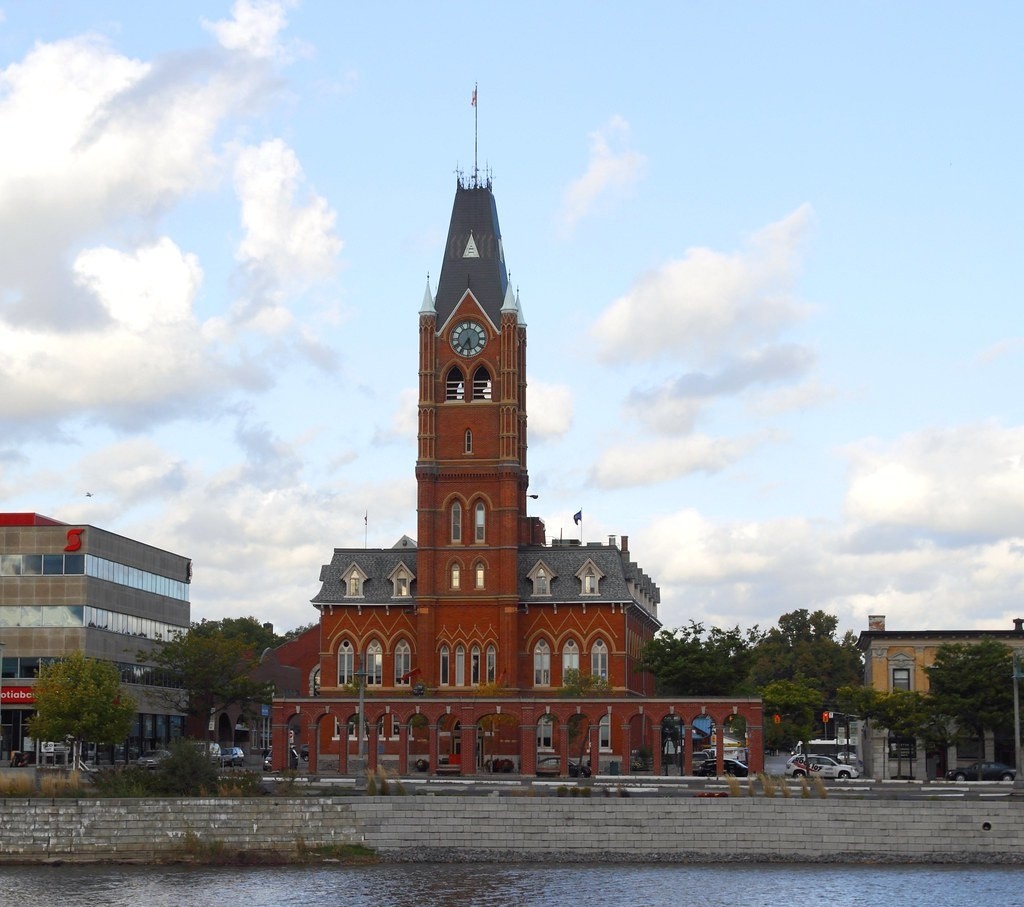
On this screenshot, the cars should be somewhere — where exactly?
[537,757,591,777]
[263,748,297,772]
[944,761,1016,781]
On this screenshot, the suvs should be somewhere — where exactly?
[301,744,309,762]
[222,746,245,768]
[187,741,222,768]
[785,753,859,780]
[689,746,749,778]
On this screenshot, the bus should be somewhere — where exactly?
[796,738,858,764]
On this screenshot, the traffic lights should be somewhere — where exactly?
[823,712,829,723]
[774,715,780,725]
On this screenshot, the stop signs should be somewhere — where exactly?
[290,731,294,738]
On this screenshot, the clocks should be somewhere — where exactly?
[449,320,488,358]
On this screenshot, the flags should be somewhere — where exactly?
[400,668,422,681]
[574,511,582,525]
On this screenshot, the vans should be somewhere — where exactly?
[136,749,172,769]
